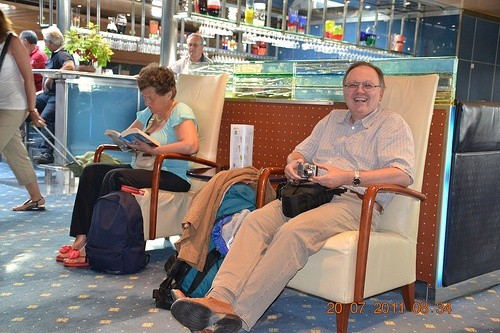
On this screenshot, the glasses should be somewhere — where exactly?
[188,43,198,47]
[344,81,380,90]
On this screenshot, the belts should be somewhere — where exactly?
[345,188,383,214]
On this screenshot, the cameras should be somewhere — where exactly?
[298,163,318,177]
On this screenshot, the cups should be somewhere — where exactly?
[71,8,80,28]
[116,13,127,34]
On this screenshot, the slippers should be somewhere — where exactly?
[56,245,89,266]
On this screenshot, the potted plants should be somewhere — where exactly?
[63,22,115,72]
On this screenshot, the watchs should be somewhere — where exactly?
[352,171,361,188]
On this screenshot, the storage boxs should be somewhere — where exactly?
[229,124,254,168]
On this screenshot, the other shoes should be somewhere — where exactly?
[169,289,243,333]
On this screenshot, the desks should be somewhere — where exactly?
[186,166,286,183]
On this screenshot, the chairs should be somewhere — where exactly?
[256,74,439,333]
[94,72,228,240]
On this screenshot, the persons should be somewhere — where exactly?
[0,9,44,211]
[34,24,75,163]
[167,32,213,72]
[172,65,415,333]
[17,31,48,92]
[55,63,200,267]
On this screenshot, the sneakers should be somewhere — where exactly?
[33,152,54,164]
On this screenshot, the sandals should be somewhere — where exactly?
[13,196,45,211]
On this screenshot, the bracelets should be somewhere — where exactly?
[29,109,37,114]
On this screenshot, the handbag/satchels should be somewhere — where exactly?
[281,179,348,218]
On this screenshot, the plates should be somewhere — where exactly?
[235,93,292,99]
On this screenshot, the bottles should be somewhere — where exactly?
[325,20,343,41]
[390,33,404,52]
[245,0,266,27]
[107,16,118,33]
[365,25,378,46]
[197,0,221,17]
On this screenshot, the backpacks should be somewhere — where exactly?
[153,183,257,311]
[85,190,151,275]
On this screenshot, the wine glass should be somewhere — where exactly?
[103,37,160,55]
[178,19,300,63]
[301,36,412,61]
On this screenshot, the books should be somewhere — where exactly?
[102,128,161,153]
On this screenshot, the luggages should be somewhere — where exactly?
[29,121,121,177]
